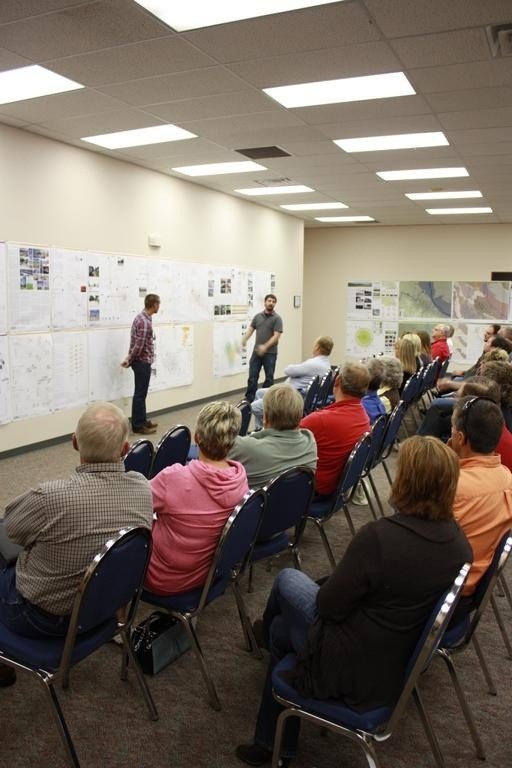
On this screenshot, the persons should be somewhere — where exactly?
[206,278,233,316]
[225,383,319,488]
[88,264,101,303]
[225,433,474,768]
[0,401,155,688]
[108,400,250,643]
[299,323,512,647]
[239,294,283,404]
[251,334,335,434]
[119,293,161,432]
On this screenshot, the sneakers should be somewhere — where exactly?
[112,622,127,643]
[392,439,405,450]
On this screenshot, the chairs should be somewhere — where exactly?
[299,351,383,421]
[270,562,470,765]
[437,536,510,761]
[324,416,385,541]
[295,435,371,573]
[189,401,249,462]
[364,402,408,521]
[122,439,153,479]
[150,426,188,480]
[122,491,267,710]
[392,355,452,448]
[195,466,316,652]
[0,525,156,768]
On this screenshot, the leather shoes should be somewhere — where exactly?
[131,420,158,435]
[236,738,292,767]
[253,618,270,650]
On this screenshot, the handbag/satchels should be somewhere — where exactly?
[131,609,193,675]
[347,477,371,506]
